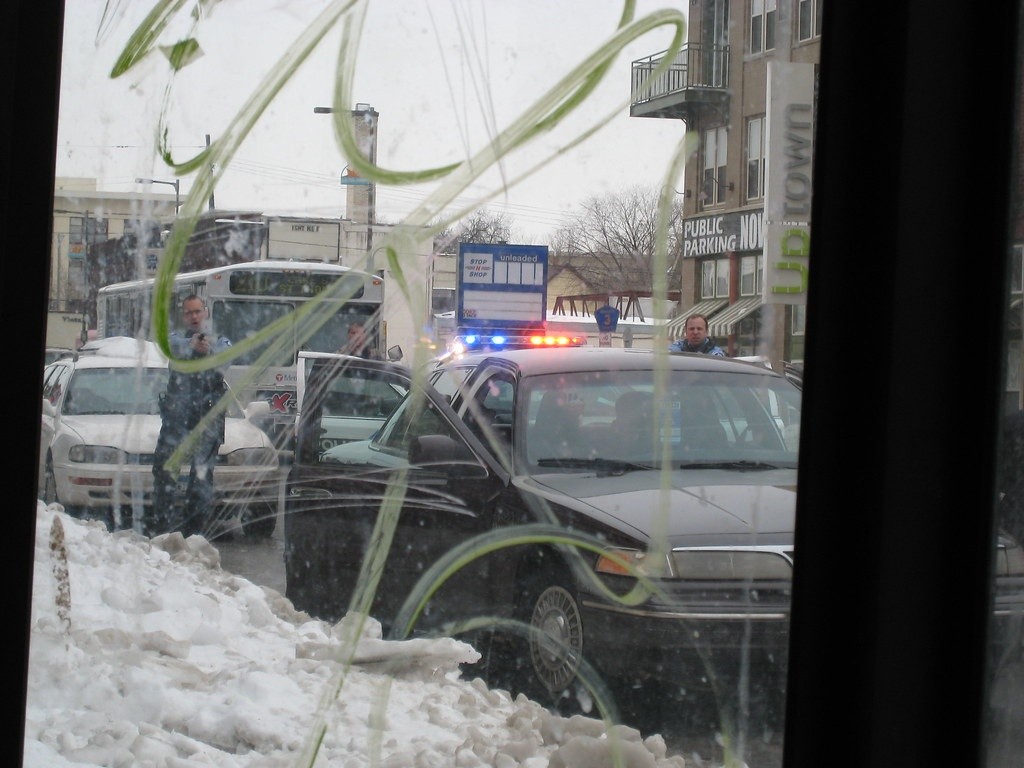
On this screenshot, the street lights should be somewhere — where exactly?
[135,177,179,222]
[314,103,379,270]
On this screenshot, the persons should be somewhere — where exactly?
[668,313,728,358]
[153,295,233,539]
[610,392,660,453]
[327,321,388,405]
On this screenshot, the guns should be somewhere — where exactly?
[199,332,205,342]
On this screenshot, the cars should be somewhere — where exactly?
[286,348,1024,732]
[39,354,278,537]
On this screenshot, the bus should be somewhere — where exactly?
[96,261,387,473]
